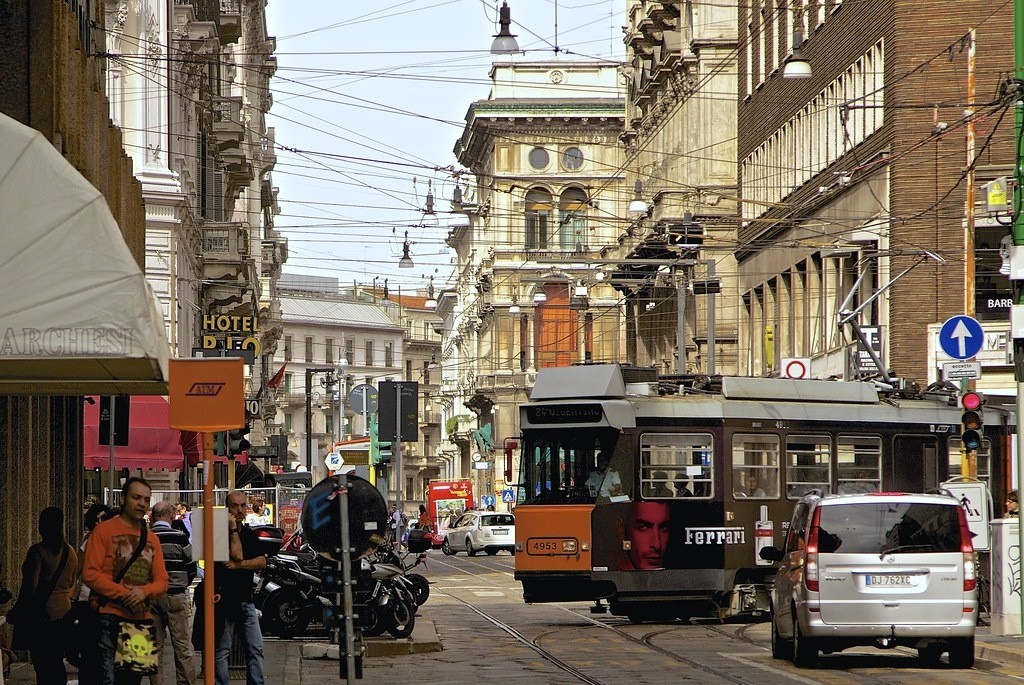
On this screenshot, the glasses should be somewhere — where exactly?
[94,516,106,522]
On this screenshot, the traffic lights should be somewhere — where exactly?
[229,406,251,454]
[213,432,226,457]
[959,393,987,453]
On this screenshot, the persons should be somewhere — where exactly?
[0,477,198,685]
[419,505,431,531]
[613,502,671,570]
[202,491,274,685]
[744,473,767,497]
[389,504,409,551]
[454,505,495,519]
[585,452,622,497]
[1003,490,1019,519]
[650,472,694,497]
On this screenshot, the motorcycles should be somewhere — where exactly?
[248,523,433,640]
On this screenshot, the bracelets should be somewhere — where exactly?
[229,529,238,533]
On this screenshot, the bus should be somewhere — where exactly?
[424,478,478,548]
[263,473,313,533]
[512,253,1023,624]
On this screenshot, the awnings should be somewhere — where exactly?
[0,112,171,395]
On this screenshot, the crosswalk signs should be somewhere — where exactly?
[936,482,988,552]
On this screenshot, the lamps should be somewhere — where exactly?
[534,271,547,300]
[426,278,437,307]
[784,11,813,78]
[418,179,439,225]
[629,168,649,211]
[510,285,520,312]
[428,355,439,369]
[399,232,415,267]
[447,178,469,225]
[380,280,392,306]
[491,2,519,54]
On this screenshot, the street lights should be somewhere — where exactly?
[304,358,351,463]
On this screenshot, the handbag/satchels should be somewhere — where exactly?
[114,610,159,676]
[61,605,99,670]
[10,610,43,651]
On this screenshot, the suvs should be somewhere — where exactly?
[758,489,981,670]
[442,512,517,557]
